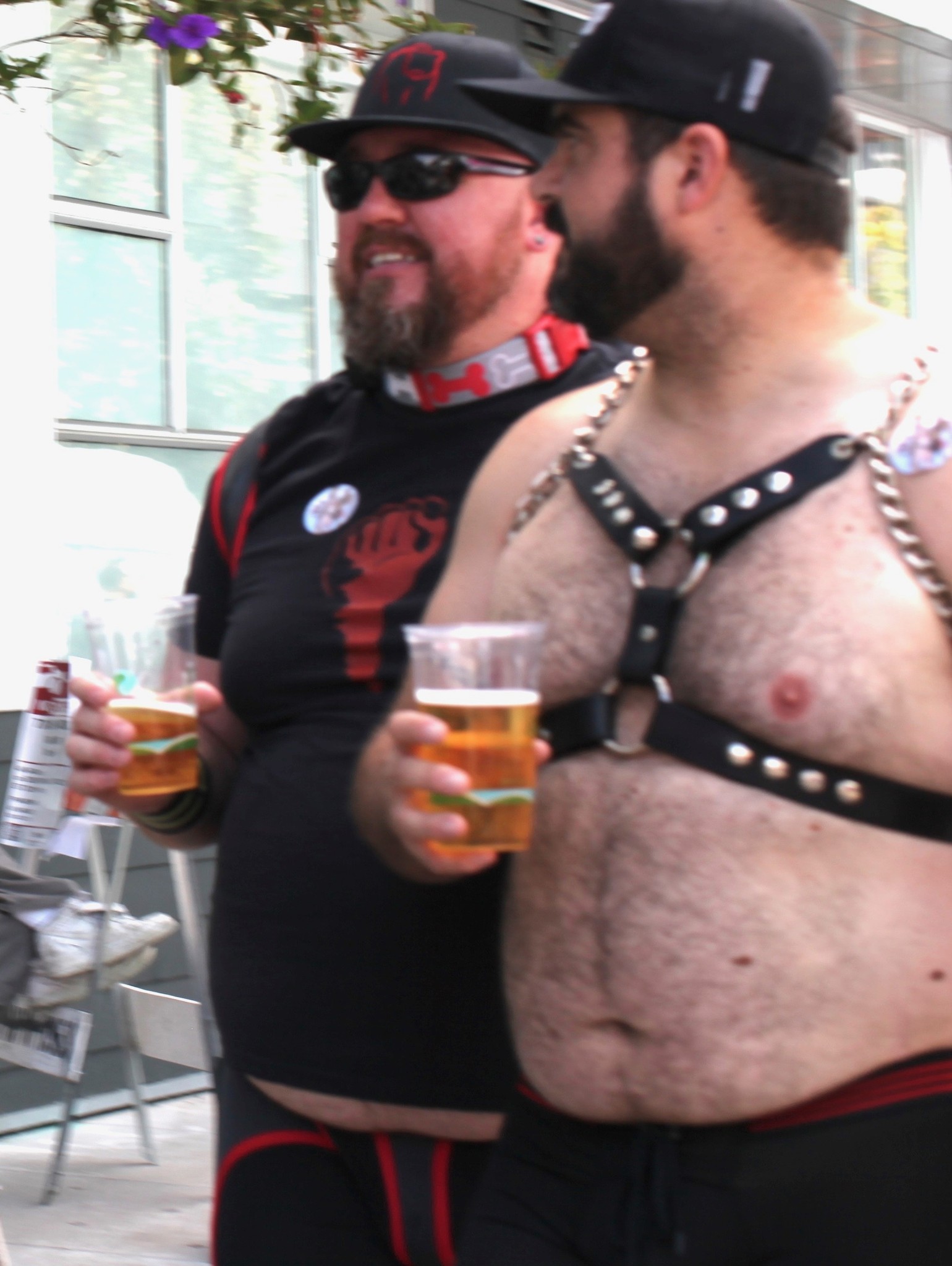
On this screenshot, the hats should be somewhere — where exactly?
[289,31,557,166]
[456,0,855,182]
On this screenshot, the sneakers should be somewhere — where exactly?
[26,945,156,1008]
[31,896,180,978]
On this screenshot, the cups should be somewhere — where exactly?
[397,617,543,855]
[83,594,199,796]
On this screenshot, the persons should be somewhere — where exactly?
[349,1,952,1266]
[62,31,653,1266]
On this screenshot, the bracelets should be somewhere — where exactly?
[131,752,212,834]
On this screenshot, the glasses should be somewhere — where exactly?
[322,145,533,213]
